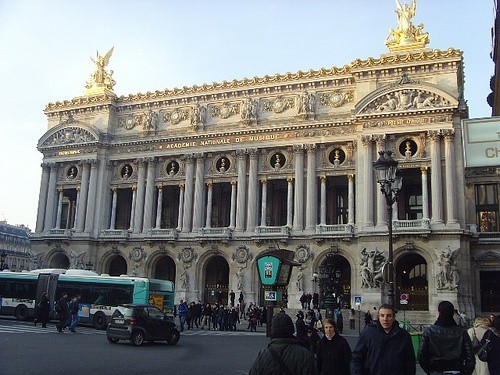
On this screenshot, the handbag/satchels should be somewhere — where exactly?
[469,327,482,355]
[70,307,76,313]
[478,329,491,361]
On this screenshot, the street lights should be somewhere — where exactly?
[372,150,400,312]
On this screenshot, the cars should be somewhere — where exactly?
[106,303,180,346]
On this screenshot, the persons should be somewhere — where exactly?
[33,292,51,328]
[180,270,190,289]
[434,247,458,290]
[248,313,315,375]
[312,319,352,375]
[174,290,268,332]
[294,269,305,291]
[141,89,436,133]
[454,308,500,375]
[280,298,288,314]
[357,247,391,288]
[294,291,380,339]
[418,301,476,375]
[349,304,416,375]
[235,266,243,290]
[54,292,82,332]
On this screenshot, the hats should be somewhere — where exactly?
[271,312,294,335]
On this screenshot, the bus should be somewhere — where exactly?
[0,268,177,330]
